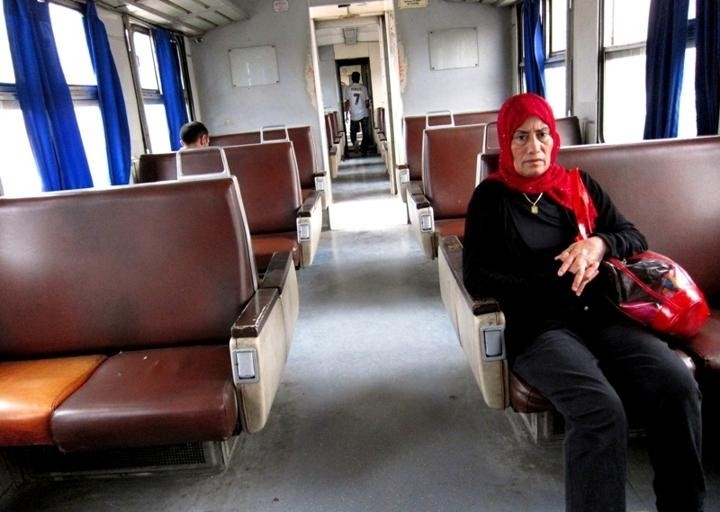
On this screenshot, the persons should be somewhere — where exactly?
[463,92,709,512]
[179,121,211,150]
[344,71,369,153]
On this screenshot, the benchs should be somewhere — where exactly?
[210,126,330,214]
[397,109,503,227]
[437,133,720,445]
[1,175,301,486]
[134,141,323,271]
[407,117,582,259]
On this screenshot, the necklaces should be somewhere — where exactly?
[523,192,545,215]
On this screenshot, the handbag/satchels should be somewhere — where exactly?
[600,251,710,337]
